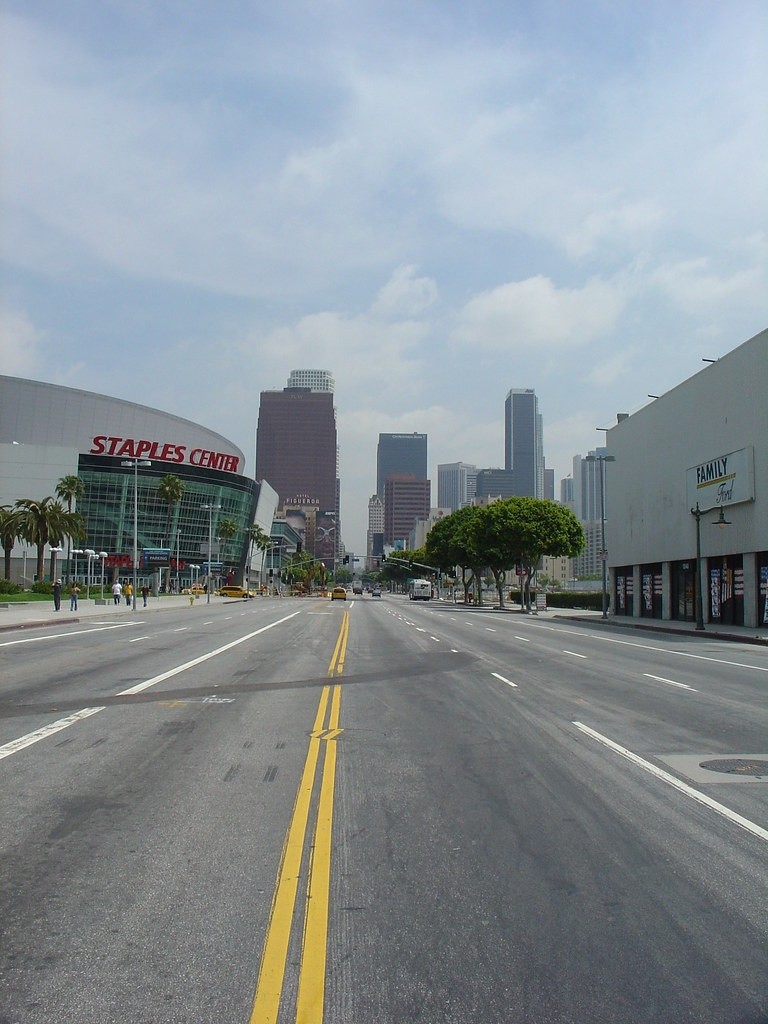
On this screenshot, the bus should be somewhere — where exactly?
[409,579,432,601]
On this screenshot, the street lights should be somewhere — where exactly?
[244,527,258,599]
[89,554,99,588]
[585,454,616,621]
[691,502,733,631]
[83,548,95,601]
[98,552,109,600]
[48,548,62,587]
[266,541,279,568]
[120,457,152,608]
[199,502,221,604]
[70,549,83,585]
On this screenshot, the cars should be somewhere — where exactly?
[351,580,384,597]
[331,586,347,601]
[217,586,255,599]
[182,587,218,596]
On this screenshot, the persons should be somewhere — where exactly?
[52,578,150,612]
[179,587,183,593]
[204,584,208,594]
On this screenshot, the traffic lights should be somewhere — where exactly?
[381,554,386,562]
[269,580,273,584]
[246,578,248,583]
[269,569,274,577]
[345,555,349,563]
[246,565,249,575]
